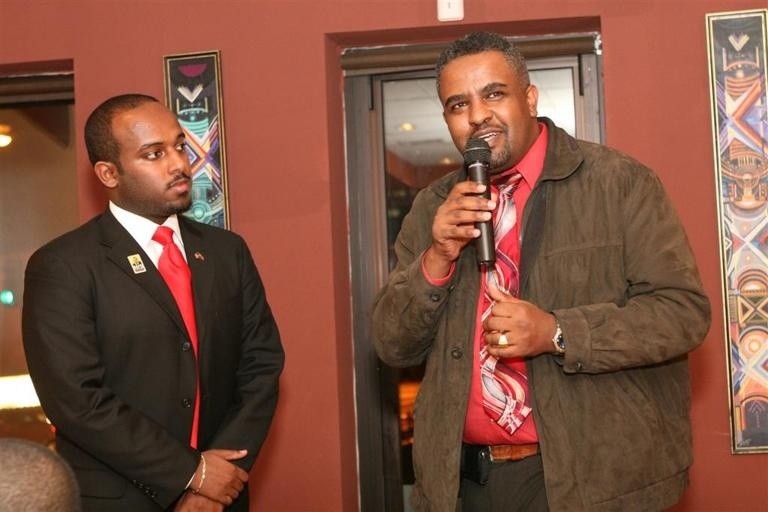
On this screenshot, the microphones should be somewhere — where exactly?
[462,135,496,269]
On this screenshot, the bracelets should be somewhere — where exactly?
[192,452,207,495]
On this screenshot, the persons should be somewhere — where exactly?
[20,93,287,511]
[369,30,713,512]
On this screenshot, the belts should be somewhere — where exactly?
[488,442,538,461]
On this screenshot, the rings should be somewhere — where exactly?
[497,335,509,345]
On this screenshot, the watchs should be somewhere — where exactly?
[551,320,566,353]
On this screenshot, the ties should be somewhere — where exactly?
[151,226,200,448]
